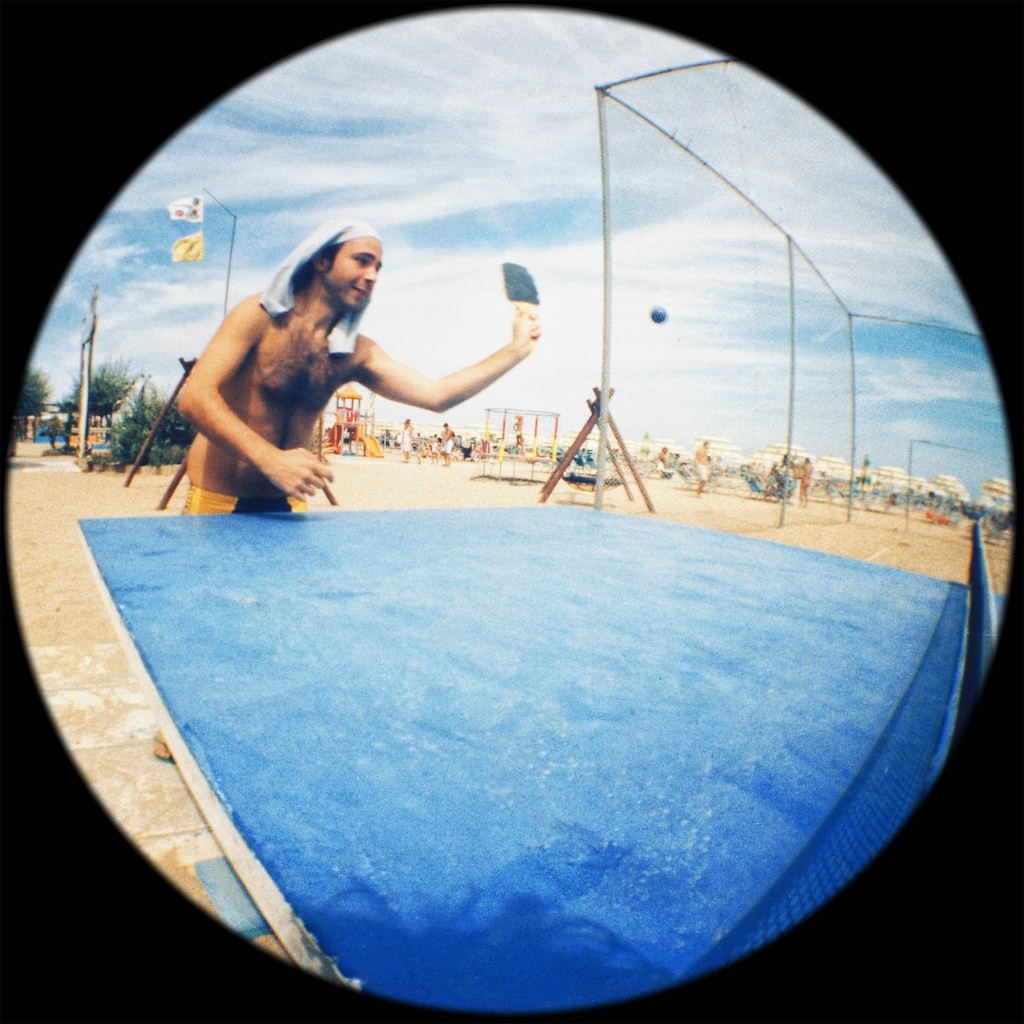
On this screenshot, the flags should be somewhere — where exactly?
[173,233,204,260]
[170,194,205,220]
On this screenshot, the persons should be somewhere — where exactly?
[576,449,597,469]
[416,434,440,464]
[694,441,709,494]
[752,455,812,507]
[401,419,413,463]
[441,423,455,467]
[154,220,540,762]
[656,447,679,478]
[513,416,524,454]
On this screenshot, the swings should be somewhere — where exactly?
[563,427,623,493]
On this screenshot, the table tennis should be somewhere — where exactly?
[651,305,669,323]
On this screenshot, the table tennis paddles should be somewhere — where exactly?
[499,262,540,332]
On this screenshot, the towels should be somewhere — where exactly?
[257,216,386,356]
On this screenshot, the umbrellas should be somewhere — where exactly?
[856,455,870,493]
[639,432,651,457]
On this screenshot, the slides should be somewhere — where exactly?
[359,434,384,457]
